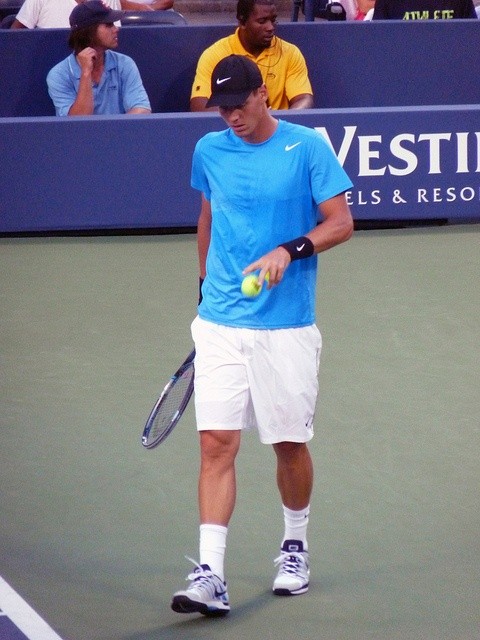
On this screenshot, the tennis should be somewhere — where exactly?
[241,275,261,298]
[264,271,270,281]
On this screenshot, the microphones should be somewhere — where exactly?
[263,33,278,48]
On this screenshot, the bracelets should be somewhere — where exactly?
[355,8,366,21]
[198,276,204,304]
[277,235,315,262]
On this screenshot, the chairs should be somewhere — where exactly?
[120,11,185,25]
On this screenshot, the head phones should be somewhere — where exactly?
[236,0,248,25]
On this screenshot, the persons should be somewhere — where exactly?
[170,54,355,616]
[46,0,152,116]
[11,1,174,29]
[190,0,315,112]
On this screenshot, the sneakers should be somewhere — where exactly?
[171,555,231,616]
[270,541,312,597]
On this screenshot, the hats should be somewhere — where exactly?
[205,54,262,108]
[69,0,129,30]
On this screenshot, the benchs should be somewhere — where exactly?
[0,18,480,121]
